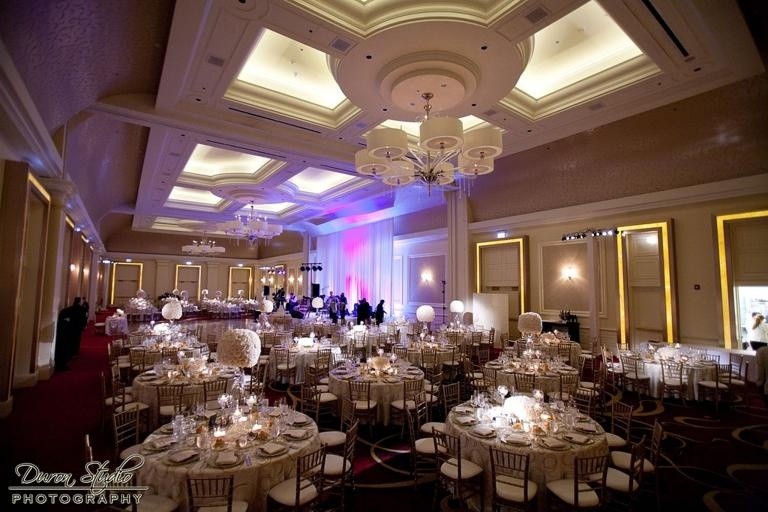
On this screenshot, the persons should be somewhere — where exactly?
[276,287,285,305]
[285,293,297,311]
[272,292,281,308]
[327,291,388,328]
[58,295,85,362]
[81,297,90,329]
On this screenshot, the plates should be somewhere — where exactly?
[567,412,609,444]
[450,403,476,426]
[258,413,312,457]
[499,433,570,452]
[141,426,199,467]
[204,447,245,468]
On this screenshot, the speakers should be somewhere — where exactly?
[312,284,319,298]
[264,286,270,296]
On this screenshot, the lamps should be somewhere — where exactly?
[181,228,226,257]
[350,90,507,200]
[266,266,287,276]
[555,222,625,245]
[298,261,324,274]
[209,198,286,250]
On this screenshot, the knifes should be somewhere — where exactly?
[245,453,253,468]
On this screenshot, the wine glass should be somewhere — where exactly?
[473,386,579,438]
[128,292,480,382]
[488,329,719,372]
[171,392,291,443]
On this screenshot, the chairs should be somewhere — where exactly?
[87,286,765,512]
